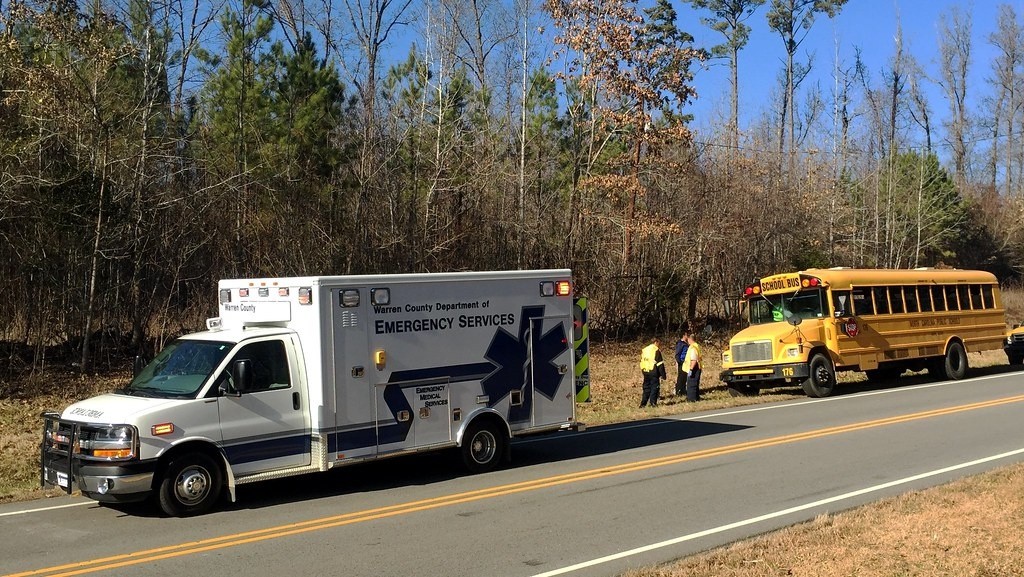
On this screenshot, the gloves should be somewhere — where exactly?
[687,370,692,377]
[662,374,666,380]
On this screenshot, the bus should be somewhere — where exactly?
[719,266,1009,398]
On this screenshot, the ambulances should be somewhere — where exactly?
[39,268,592,518]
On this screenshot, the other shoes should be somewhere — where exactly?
[639,403,645,408]
[651,403,656,406]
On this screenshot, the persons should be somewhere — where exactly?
[681,334,703,402]
[639,338,666,408]
[675,330,689,395]
[769,297,789,321]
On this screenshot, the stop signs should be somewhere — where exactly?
[846,317,859,337]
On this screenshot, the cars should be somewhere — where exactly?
[1003,322,1024,365]
